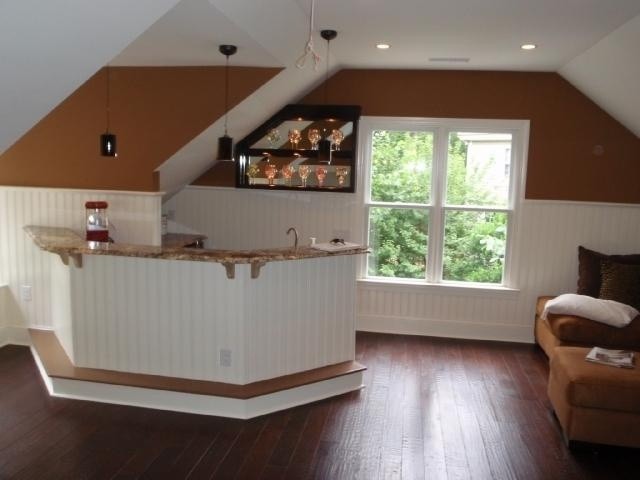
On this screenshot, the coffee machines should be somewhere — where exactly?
[84,200,116,243]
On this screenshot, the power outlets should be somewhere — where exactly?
[22,285,32,302]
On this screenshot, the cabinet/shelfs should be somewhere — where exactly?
[234,103,361,194]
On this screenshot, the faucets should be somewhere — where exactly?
[285,227,299,254]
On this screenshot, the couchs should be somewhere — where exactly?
[547,346,640,451]
[534,295,640,361]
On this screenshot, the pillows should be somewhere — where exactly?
[538,290,640,330]
[596,254,639,310]
[577,244,640,295]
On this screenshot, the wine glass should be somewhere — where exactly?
[248,164,348,188]
[267,127,345,150]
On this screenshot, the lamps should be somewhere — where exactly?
[318,29,337,164]
[100,63,117,157]
[214,44,238,163]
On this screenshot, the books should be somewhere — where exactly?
[583,345,636,369]
[313,240,361,253]
